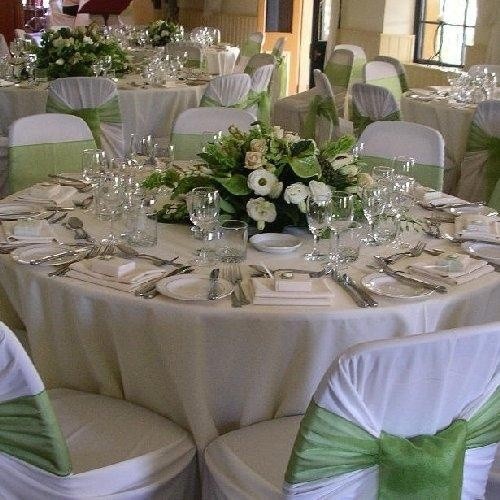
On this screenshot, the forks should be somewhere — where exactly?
[429,200,488,209]
[222,264,250,308]
[114,251,179,268]
[48,243,114,279]
[379,240,428,266]
[442,232,500,245]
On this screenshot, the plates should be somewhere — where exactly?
[8,244,66,265]
[361,269,436,299]
[248,233,304,254]
[58,172,86,186]
[430,197,485,214]
[0,201,40,220]
[155,273,234,302]
[459,241,500,263]
[448,203,499,220]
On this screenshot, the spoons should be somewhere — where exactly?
[249,263,321,276]
[114,242,187,267]
[68,217,91,239]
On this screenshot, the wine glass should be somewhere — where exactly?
[388,154,415,244]
[90,54,112,79]
[302,192,331,262]
[132,46,189,88]
[82,149,141,222]
[324,191,351,265]
[368,166,394,204]
[99,23,150,48]
[1,40,37,85]
[189,24,217,50]
[183,186,221,266]
[451,63,497,103]
[360,185,385,247]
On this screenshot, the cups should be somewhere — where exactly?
[109,203,160,246]
[198,130,224,156]
[127,132,176,172]
[215,220,248,266]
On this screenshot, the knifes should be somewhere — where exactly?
[30,245,99,265]
[143,268,196,300]
[328,268,377,309]
[47,174,82,182]
[206,266,221,300]
[134,264,194,297]
